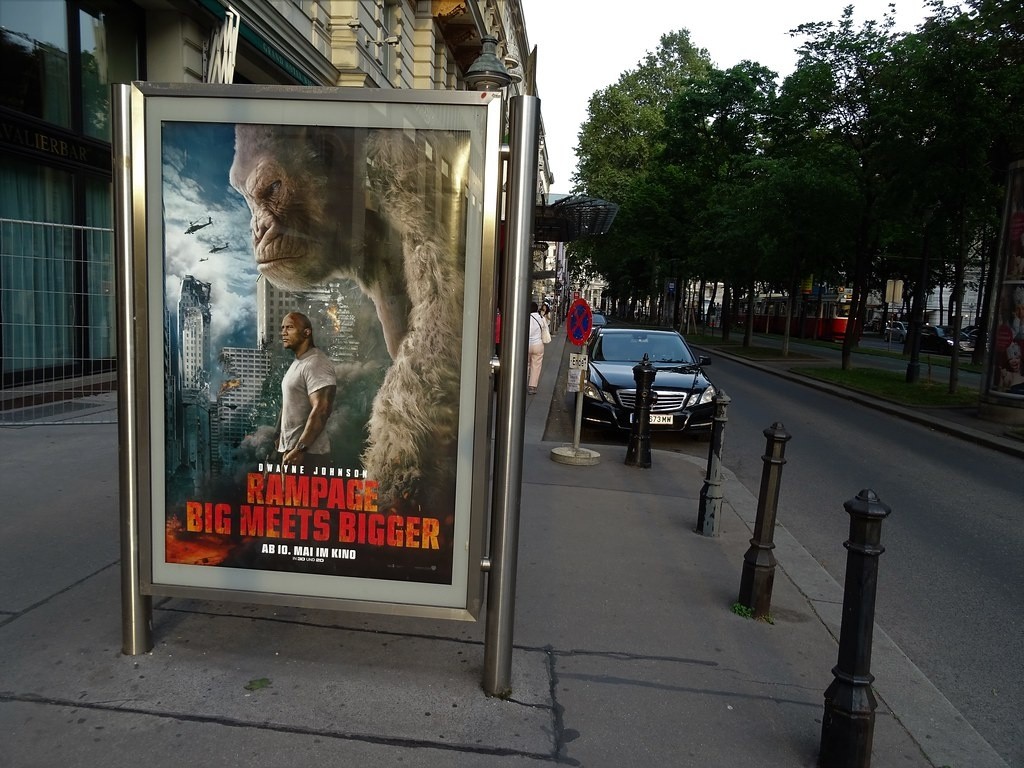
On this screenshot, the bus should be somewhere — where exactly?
[738,290,868,342]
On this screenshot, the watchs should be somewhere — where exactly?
[296,442,308,451]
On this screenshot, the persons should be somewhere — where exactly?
[269,312,337,469]
[709,302,720,327]
[998,287,1024,393]
[494,302,550,394]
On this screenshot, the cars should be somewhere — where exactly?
[591,310,608,329]
[920,324,971,355]
[961,325,976,348]
[883,320,909,343]
[573,328,717,435]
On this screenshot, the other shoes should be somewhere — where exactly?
[528,387,537,395]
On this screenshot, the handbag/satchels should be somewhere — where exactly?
[541,316,552,344]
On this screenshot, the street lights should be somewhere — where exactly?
[179,399,240,501]
[968,302,975,325]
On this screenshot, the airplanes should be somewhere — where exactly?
[208,242,230,254]
[198,257,209,262]
[183,215,214,236]
